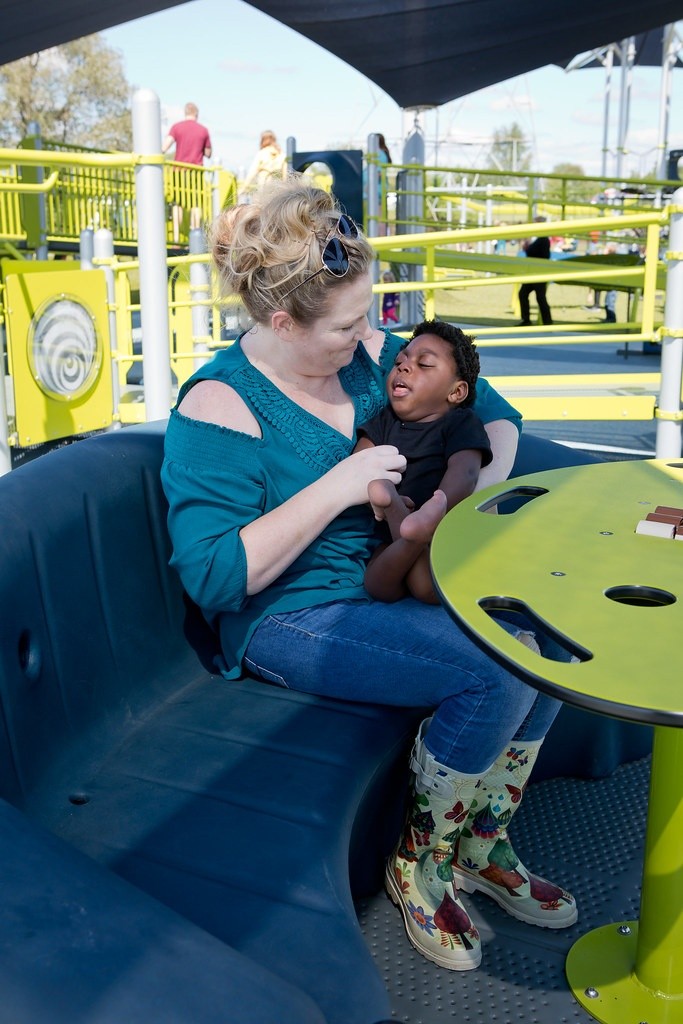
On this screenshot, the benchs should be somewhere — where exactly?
[1,419,657,1024]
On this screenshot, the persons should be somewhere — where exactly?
[602,244,617,324]
[382,270,401,326]
[158,181,580,971]
[352,320,494,606]
[513,214,553,327]
[253,130,283,186]
[488,219,668,271]
[161,102,212,209]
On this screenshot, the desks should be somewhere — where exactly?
[425,457,682,1022]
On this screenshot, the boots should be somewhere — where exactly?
[385,713,494,970]
[451,736,578,928]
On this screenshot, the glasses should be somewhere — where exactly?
[277,215,358,302]
